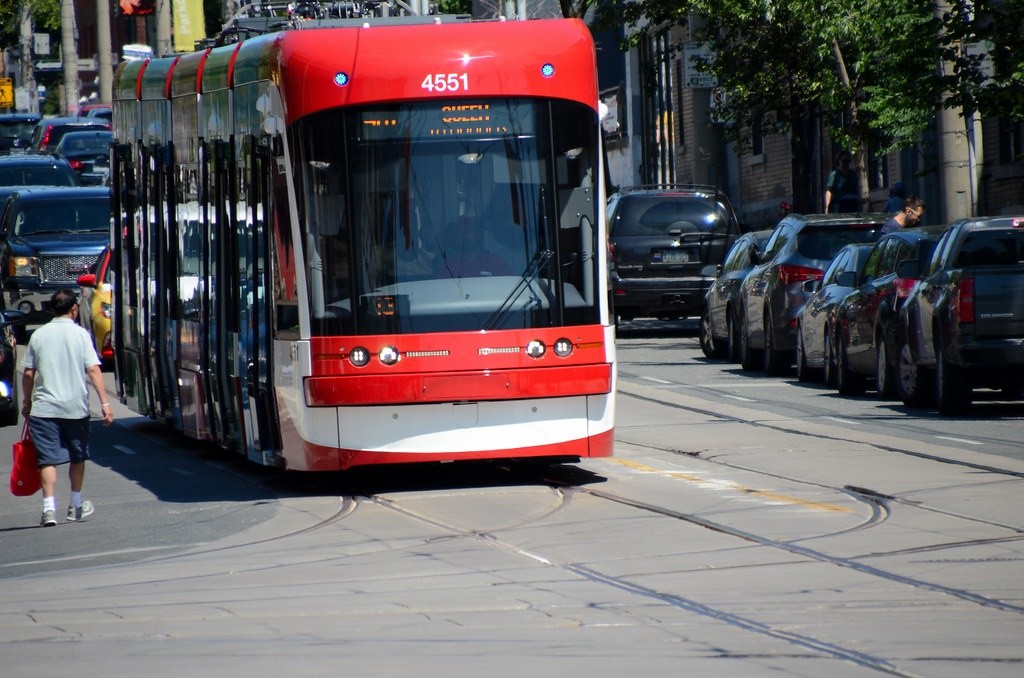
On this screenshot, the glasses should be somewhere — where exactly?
[906,207,923,219]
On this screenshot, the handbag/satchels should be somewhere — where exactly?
[9,419,43,497]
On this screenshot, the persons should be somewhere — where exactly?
[825,150,860,216]
[882,193,905,213]
[22,287,114,527]
[879,196,927,238]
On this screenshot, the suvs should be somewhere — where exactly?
[604,183,743,340]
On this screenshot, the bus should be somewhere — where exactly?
[110,0,630,479]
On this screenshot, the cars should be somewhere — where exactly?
[893,215,1024,416]
[697,214,888,377]
[0,105,111,430]
[832,222,949,404]
[790,241,887,387]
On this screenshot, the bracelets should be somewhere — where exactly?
[100,403,110,407]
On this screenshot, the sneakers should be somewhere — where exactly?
[39,509,57,528]
[67,501,95,521]
